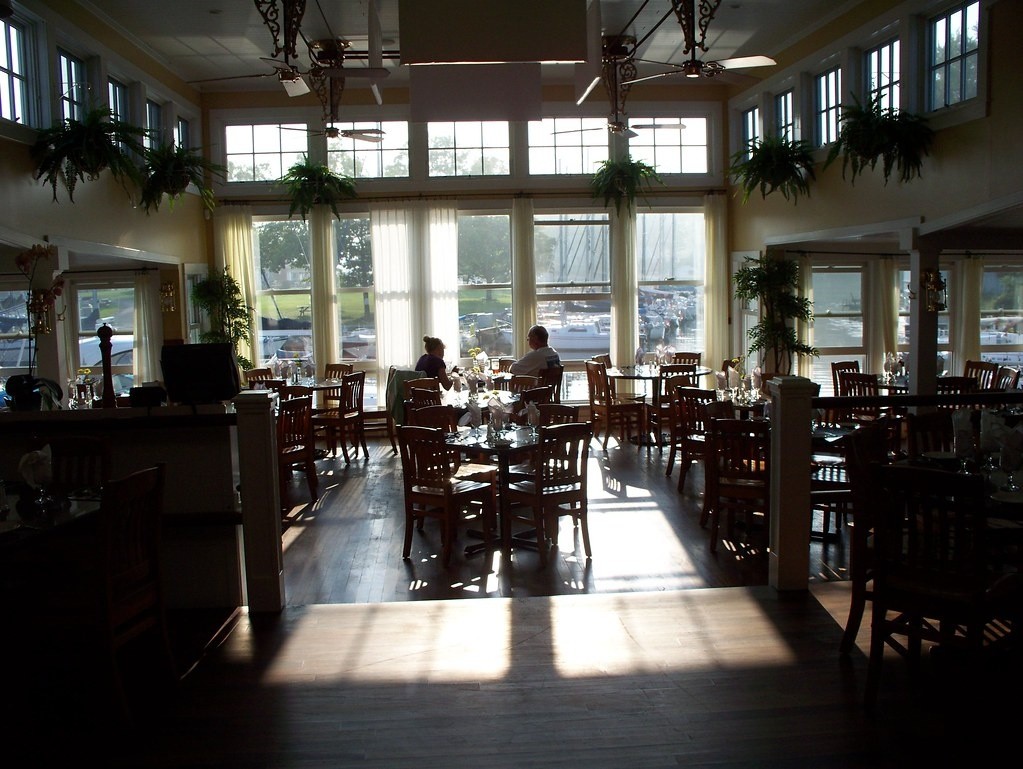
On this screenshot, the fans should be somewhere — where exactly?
[186,0,390,98]
[277,61,385,143]
[620,0,777,86]
[549,35,687,139]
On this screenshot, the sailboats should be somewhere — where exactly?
[475,212,699,364]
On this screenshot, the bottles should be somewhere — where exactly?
[492,359,499,374]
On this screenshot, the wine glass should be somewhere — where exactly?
[998,447,1021,492]
[528,406,539,438]
[729,378,740,398]
[753,377,762,399]
[470,416,482,437]
[491,417,502,439]
[717,380,727,398]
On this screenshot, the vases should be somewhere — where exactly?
[5,374,38,411]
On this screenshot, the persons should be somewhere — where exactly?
[509,325,560,391]
[415,336,459,391]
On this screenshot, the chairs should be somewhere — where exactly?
[38,353,1023,724]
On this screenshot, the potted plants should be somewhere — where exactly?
[722,128,817,208]
[587,153,670,217]
[28,87,161,205]
[820,90,936,189]
[729,251,821,376]
[138,139,227,217]
[268,151,360,223]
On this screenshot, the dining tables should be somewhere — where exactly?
[603,363,711,379]
[0,494,101,534]
[882,450,1023,520]
[282,376,356,390]
[442,421,557,574]
[856,376,908,390]
[809,428,848,469]
[714,388,772,411]
[273,392,308,419]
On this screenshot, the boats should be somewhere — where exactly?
[828,280,1022,375]
[275,333,375,364]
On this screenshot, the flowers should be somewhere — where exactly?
[16,245,66,375]
